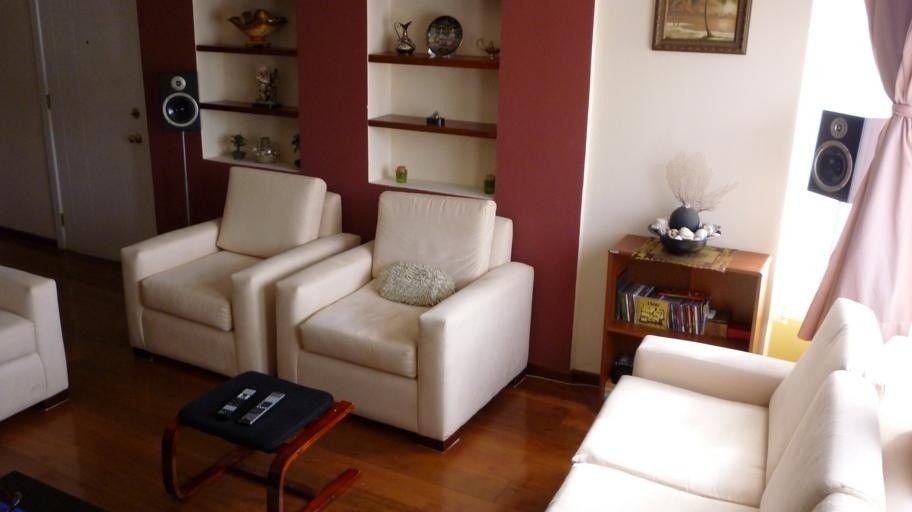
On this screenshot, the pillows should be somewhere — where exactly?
[376,262,457,308]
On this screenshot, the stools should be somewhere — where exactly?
[159,370,362,510]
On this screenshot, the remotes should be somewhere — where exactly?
[216,385,258,419]
[238,390,288,426]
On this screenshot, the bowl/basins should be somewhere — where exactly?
[659,236,706,254]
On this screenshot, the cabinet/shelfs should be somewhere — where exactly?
[598,235,776,411]
[368,48,498,141]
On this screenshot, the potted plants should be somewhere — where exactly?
[230,132,248,159]
[291,133,302,169]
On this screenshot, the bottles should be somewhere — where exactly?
[396,165,408,182]
[483,174,496,194]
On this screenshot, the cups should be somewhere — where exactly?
[259,137,270,151]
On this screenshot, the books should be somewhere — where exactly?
[615,283,712,336]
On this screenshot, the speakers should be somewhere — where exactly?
[159,68,201,131]
[807,110,888,204]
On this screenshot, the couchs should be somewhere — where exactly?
[0,262,69,430]
[120,167,359,378]
[545,296,885,512]
[277,188,532,451]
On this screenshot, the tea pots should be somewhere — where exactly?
[393,21,416,55]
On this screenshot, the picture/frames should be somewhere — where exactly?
[195,41,299,120]
[651,0,754,55]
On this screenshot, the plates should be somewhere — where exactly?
[426,14,464,57]
